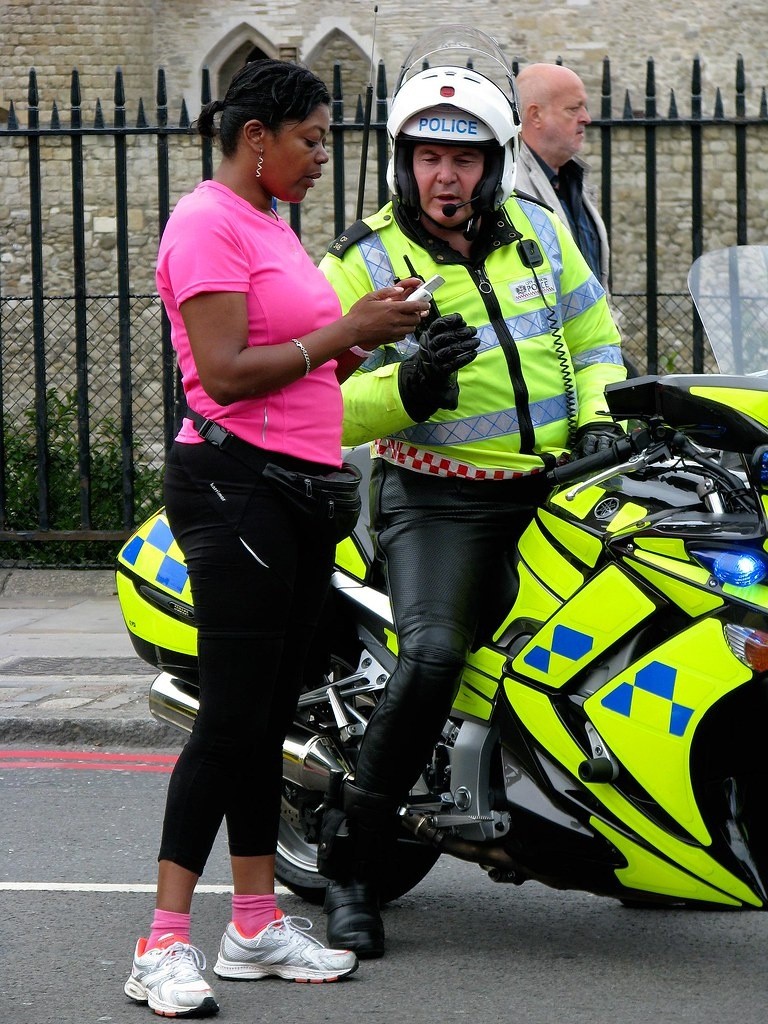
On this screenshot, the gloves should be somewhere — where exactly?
[398,313,480,422]
[564,422,624,465]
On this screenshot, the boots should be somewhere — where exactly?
[324,780,409,959]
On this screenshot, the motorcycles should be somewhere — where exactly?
[114,244,767,911]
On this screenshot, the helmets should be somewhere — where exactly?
[387,64,521,210]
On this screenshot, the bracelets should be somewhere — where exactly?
[290,338,310,380]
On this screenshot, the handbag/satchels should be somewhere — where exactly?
[185,407,361,542]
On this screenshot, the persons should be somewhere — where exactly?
[123,60,431,1020]
[317,19,629,962]
[512,64,612,303]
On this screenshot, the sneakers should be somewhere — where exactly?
[213,908,358,984]
[124,936,219,1017]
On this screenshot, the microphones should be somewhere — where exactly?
[441,195,481,217]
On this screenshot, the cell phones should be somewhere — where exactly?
[405,275,446,303]
